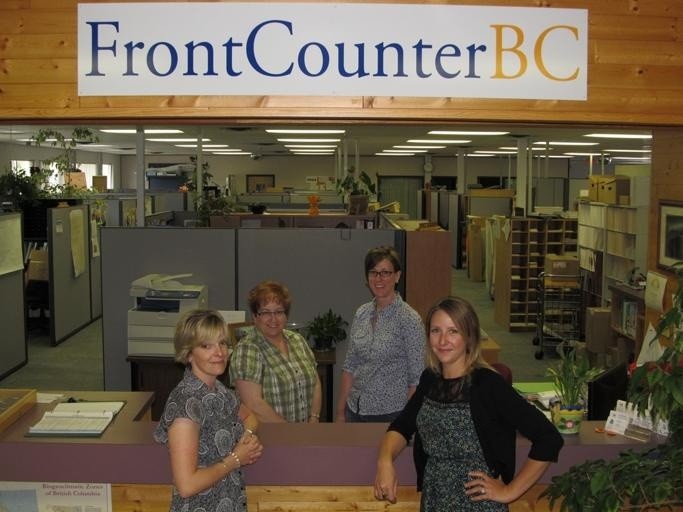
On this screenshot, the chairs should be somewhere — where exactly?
[24,259,49,335]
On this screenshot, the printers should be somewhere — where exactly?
[128,274,208,357]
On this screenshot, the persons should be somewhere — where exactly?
[334,246,428,422]
[153,303,265,511]
[370,294,564,511]
[228,278,323,423]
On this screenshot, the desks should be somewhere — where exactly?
[480,328,500,365]
[126,347,336,422]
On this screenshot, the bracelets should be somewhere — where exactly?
[229,451,241,469]
[218,458,231,478]
[308,412,320,419]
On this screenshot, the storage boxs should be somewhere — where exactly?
[599,177,631,203]
[589,176,598,201]
[545,256,579,282]
[25,261,49,280]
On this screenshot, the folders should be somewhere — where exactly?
[24,401,126,437]
[511,382,569,402]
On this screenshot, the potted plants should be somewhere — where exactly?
[305,309,349,352]
[248,202,268,214]
[545,340,601,434]
[25,127,103,191]
[336,171,376,215]
[14,166,50,196]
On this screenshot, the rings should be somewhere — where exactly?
[479,486,485,494]
[383,492,389,500]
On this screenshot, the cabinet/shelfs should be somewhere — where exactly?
[533,272,583,359]
[576,199,649,337]
[396,220,451,323]
[488,215,578,332]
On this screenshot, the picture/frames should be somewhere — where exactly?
[246,175,274,192]
[656,199,683,274]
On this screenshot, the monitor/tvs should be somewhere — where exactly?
[22,199,84,242]
[588,361,627,420]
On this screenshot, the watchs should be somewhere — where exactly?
[246,428,256,436]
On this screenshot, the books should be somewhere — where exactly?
[573,201,637,279]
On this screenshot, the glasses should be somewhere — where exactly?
[368,270,394,277]
[255,310,286,318]
